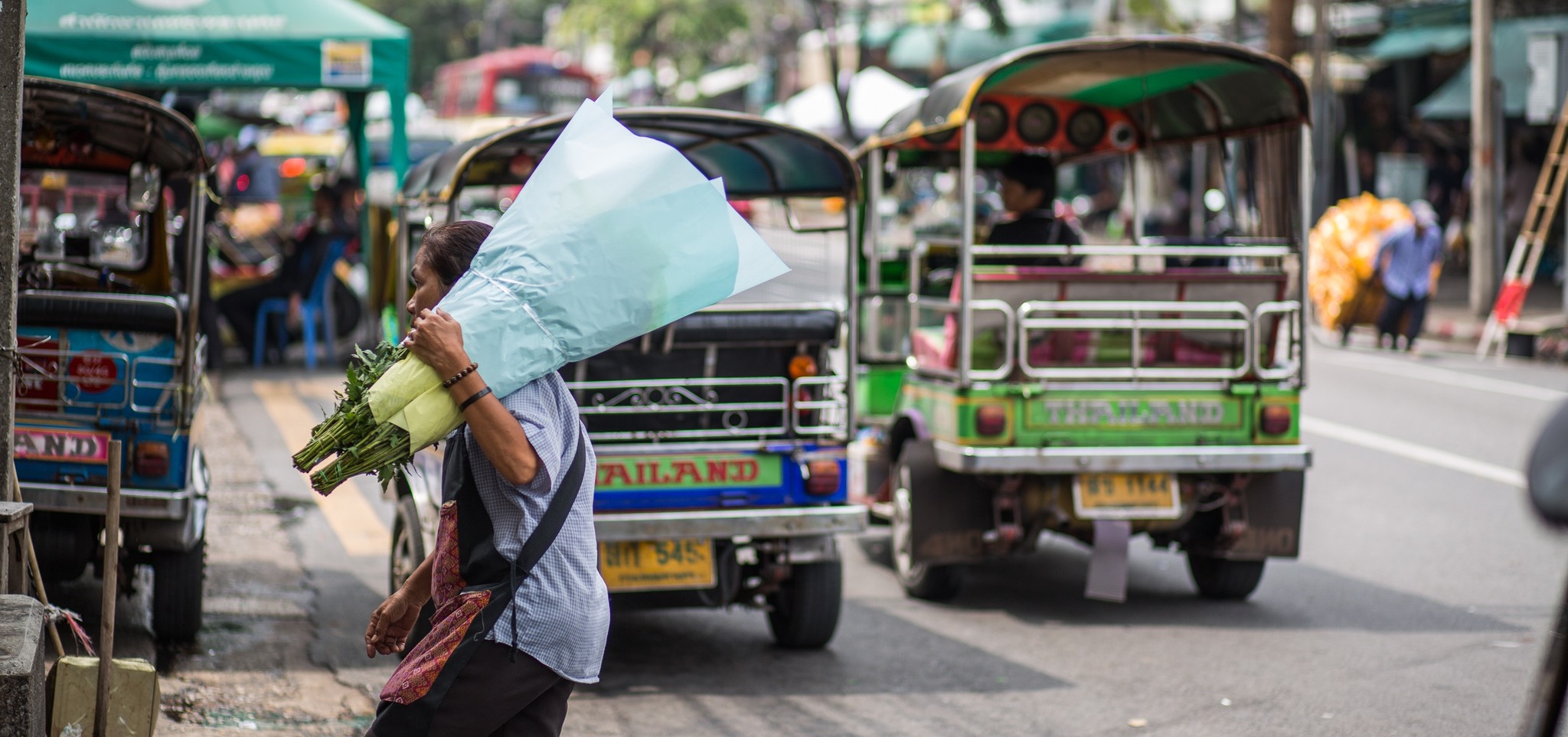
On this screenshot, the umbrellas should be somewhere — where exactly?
[1360,0,1568,126]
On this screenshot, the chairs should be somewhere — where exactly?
[251,243,345,372]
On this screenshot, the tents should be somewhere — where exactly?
[23,0,413,349]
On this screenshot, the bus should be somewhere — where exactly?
[215,44,608,353]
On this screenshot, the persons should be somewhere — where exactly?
[365,222,610,737]
[23,137,353,371]
[884,150,1236,270]
[1373,199,1443,350]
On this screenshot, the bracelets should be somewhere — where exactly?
[458,387,492,412]
[443,362,478,388]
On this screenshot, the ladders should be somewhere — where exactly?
[1478,100,1568,358]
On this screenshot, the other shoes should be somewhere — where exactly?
[266,349,285,365]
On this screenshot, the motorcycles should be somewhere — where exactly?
[855,32,1315,601]
[374,102,873,654]
[7,72,223,648]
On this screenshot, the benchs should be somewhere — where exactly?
[911,267,1292,395]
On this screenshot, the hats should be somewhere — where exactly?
[979,153,1054,191]
[1413,200,1436,228]
